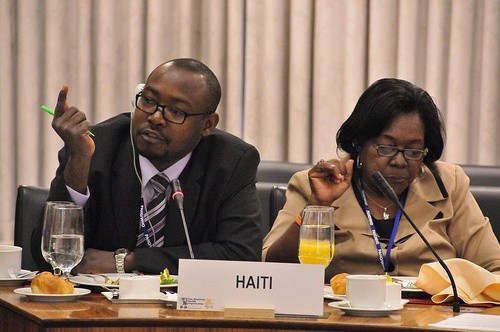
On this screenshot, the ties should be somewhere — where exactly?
[135,173,171,248]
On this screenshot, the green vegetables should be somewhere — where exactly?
[160,271,178,285]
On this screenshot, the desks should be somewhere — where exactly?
[0,284,500,332]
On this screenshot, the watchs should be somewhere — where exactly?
[112,248,129,274]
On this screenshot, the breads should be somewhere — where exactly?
[331,273,352,295]
[30,271,74,294]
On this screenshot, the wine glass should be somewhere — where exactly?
[50,205,85,279]
[40,201,75,278]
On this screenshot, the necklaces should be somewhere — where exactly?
[366,192,395,220]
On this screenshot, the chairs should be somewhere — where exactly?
[268,186,288,230]
[12,182,49,271]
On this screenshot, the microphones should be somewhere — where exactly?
[370,171,460,313]
[172,179,194,259]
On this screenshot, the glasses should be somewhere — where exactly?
[135,91,206,125]
[372,138,428,161]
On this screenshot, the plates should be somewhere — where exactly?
[391,277,423,292]
[99,291,177,304]
[0,270,37,284]
[13,287,91,301]
[328,301,404,316]
[70,274,178,289]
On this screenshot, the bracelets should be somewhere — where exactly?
[295,214,304,227]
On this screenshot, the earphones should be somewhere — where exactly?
[130,84,145,107]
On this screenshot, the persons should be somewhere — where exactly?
[261,78,500,278]
[31,58,262,275]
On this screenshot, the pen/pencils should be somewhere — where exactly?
[41,105,96,137]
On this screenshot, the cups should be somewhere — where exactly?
[346,275,387,307]
[0,244,22,278]
[299,206,335,268]
[119,275,159,299]
